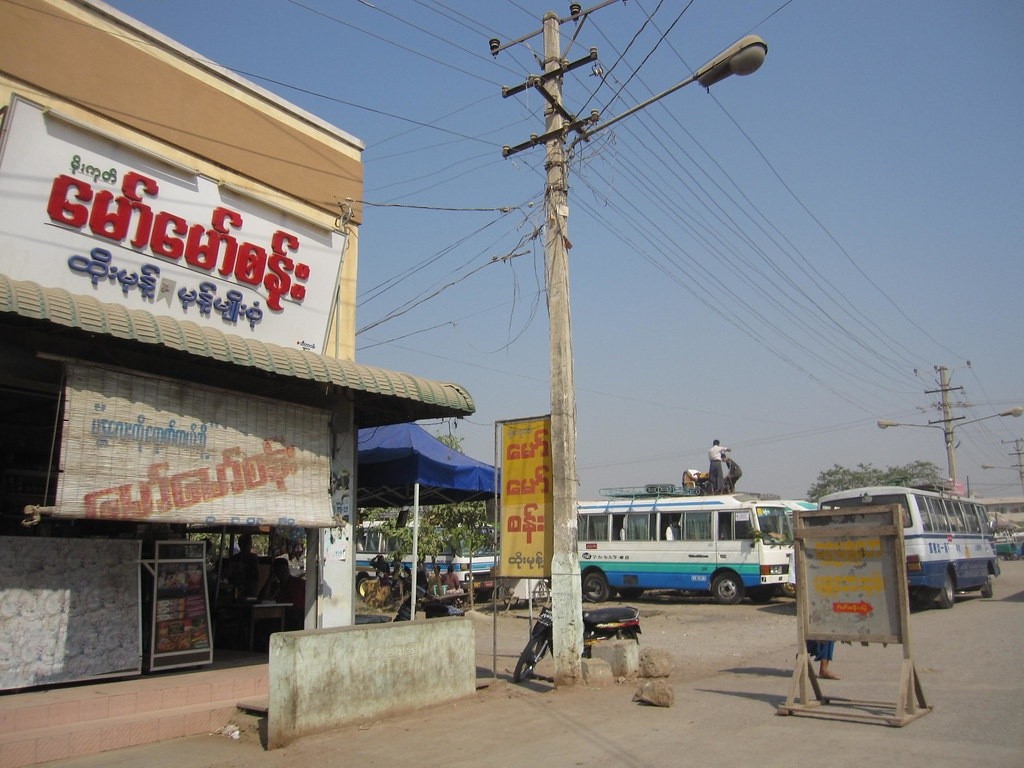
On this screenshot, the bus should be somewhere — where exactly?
[356,516,501,603]
[763,499,841,599]
[577,482,796,606]
[817,484,1001,610]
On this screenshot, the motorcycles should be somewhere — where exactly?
[513,578,642,684]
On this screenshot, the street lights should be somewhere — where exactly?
[876,406,1024,482]
[551,34,769,694]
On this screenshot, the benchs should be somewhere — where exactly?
[620,527,680,540]
[900,510,965,531]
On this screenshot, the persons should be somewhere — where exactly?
[707,440,731,495]
[232,535,259,565]
[370,555,467,608]
[807,639,842,681]
[721,453,743,494]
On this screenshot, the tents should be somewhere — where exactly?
[357,422,533,626]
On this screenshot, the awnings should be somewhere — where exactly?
[0,275,475,428]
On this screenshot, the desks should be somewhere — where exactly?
[213,596,294,652]
